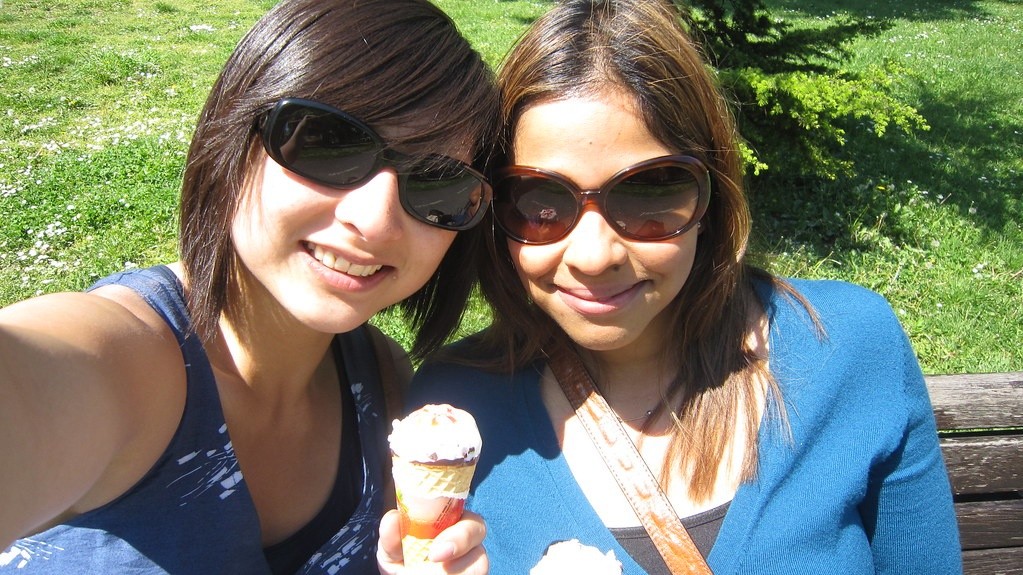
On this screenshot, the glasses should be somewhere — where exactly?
[253,97,494,232]
[490,155,711,246]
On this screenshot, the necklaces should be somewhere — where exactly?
[619,410,653,424]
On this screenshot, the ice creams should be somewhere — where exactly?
[387,403,483,575]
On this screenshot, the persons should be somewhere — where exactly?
[1,0,504,575]
[406,0,965,575]
